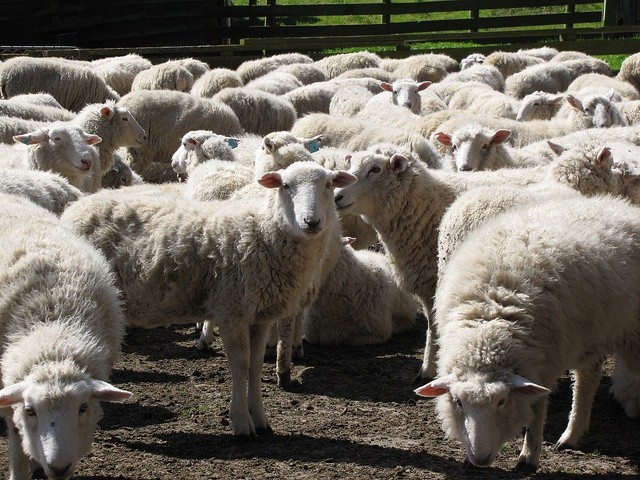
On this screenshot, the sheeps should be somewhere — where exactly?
[279,63,326,84]
[392,60,446,82]
[171,129,234,352]
[437,126,640,172]
[0,99,146,180]
[84,52,153,98]
[233,130,342,391]
[0,168,85,218]
[456,64,505,93]
[330,67,390,85]
[560,58,612,80]
[515,44,560,63]
[0,119,102,180]
[131,64,195,93]
[615,52,640,98]
[237,52,314,85]
[384,52,459,73]
[333,142,546,386]
[483,50,546,79]
[58,160,359,436]
[516,87,623,122]
[244,71,304,95]
[0,99,133,189]
[341,78,384,95]
[414,193,640,476]
[565,72,640,102]
[425,71,463,107]
[366,78,432,116]
[0,192,134,480]
[120,91,242,180]
[190,67,244,97]
[0,56,122,131]
[418,87,448,114]
[448,79,514,119]
[292,111,437,251]
[329,85,373,118]
[312,49,382,81]
[167,57,212,81]
[567,90,640,132]
[435,140,613,450]
[283,81,340,118]
[550,51,588,62]
[461,53,487,70]
[504,62,571,99]
[211,86,298,138]
[253,130,334,360]
[302,234,417,348]
